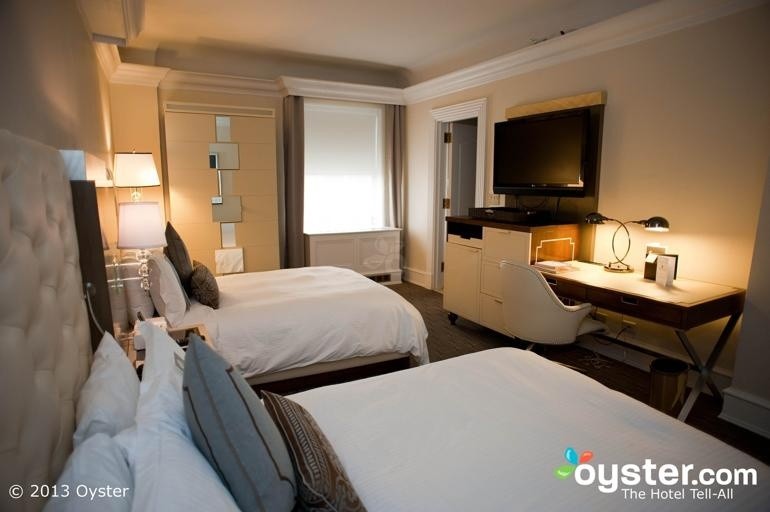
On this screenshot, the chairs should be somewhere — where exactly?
[499,258,607,352]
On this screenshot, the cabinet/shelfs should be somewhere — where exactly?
[304,226,404,286]
[479,223,581,341]
[443,215,482,327]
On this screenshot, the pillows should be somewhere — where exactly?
[164,222,193,286]
[73,331,141,453]
[192,261,220,309]
[261,389,364,511]
[108,255,185,328]
[135,322,240,511]
[40,432,133,512]
[183,331,299,512]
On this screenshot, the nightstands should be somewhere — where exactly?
[113,323,215,378]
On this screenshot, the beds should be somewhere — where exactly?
[106,264,433,386]
[42,329,769,510]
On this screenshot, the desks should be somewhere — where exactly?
[533,258,747,422]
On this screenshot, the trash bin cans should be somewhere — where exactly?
[648,357,691,419]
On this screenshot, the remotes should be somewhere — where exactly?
[176,336,205,346]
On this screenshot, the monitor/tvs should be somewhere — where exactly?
[493,104,605,198]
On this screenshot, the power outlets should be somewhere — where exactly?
[621,317,636,334]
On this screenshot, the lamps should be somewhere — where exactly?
[116,200,169,301]
[583,211,670,272]
[112,148,160,200]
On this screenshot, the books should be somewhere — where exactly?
[531,258,581,275]
